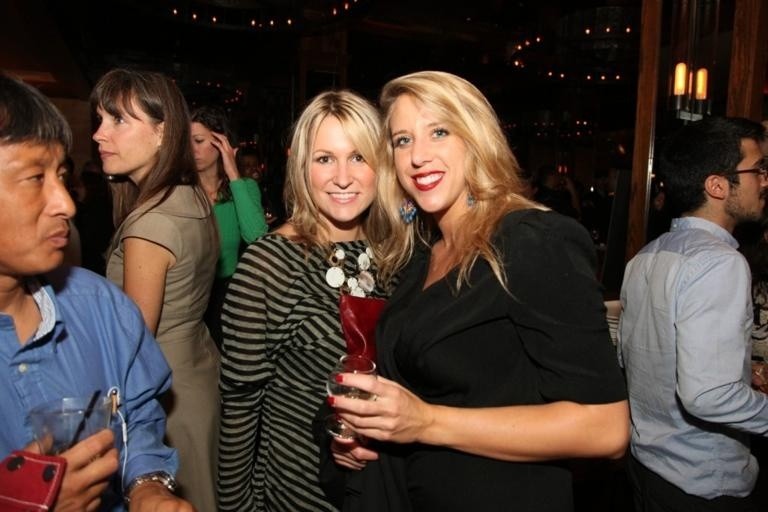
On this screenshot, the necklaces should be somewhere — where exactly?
[319,232,380,298]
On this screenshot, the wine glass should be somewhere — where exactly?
[320,354,376,437]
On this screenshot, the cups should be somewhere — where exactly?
[27,397,111,467]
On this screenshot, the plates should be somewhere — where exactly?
[604,300,619,347]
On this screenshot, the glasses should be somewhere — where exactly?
[720,166,766,180]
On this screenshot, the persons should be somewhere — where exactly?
[2,60,293,511]
[504,88,767,512]
[314,66,634,507]
[216,87,383,510]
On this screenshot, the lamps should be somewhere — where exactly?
[669,59,711,129]
